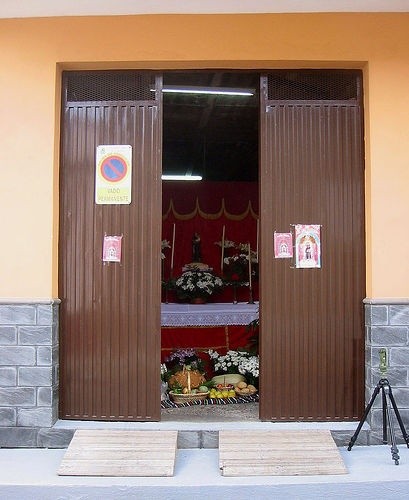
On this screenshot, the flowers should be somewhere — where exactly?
[160,343,259,385]
[215,239,259,276]
[172,268,224,299]
[161,237,171,260]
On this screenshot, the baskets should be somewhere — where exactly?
[169,371,210,403]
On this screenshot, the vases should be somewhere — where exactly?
[192,296,205,304]
[231,273,240,281]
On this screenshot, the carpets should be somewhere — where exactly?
[161,390,259,408]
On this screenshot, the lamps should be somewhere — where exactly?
[147,82,257,96]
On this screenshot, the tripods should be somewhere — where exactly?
[347,371,409,466]
